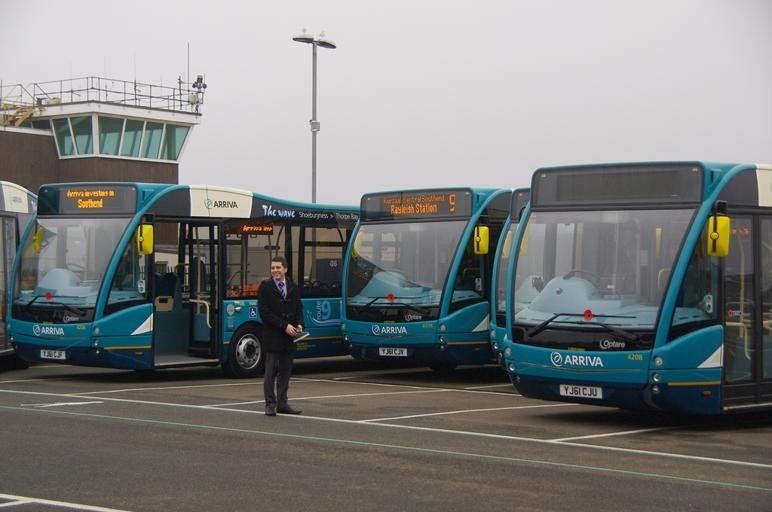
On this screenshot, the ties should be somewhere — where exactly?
[279,283,285,298]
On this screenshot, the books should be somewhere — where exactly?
[283,329,310,343]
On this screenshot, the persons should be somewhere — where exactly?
[257,256,305,416]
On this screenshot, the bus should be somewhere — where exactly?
[6,181,361,381]
[338,186,514,378]
[0,180,39,370]
[502,159,771,424]
[487,200,522,376]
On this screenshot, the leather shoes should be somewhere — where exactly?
[265,406,276,416]
[278,407,302,413]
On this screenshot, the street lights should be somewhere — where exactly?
[291,29,334,282]
